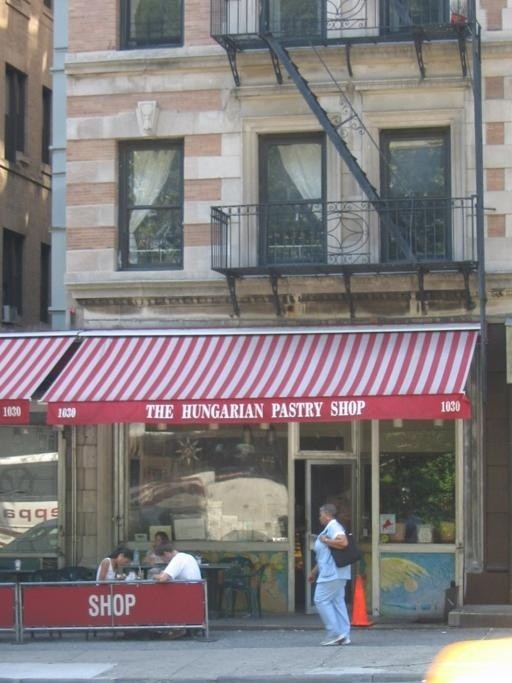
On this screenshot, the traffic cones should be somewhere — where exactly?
[349,574,375,626]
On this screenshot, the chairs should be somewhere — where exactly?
[0,556,270,641]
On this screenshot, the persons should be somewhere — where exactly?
[306,503,353,646]
[151,541,203,639]
[94,543,140,638]
[143,531,173,579]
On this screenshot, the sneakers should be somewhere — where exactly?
[320,632,351,647]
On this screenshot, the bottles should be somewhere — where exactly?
[133,547,140,566]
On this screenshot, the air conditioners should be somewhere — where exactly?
[3,304,18,322]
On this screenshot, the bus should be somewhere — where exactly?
[0,446,290,575]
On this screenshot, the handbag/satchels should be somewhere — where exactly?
[326,519,362,568]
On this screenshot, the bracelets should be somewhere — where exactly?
[323,538,328,544]
